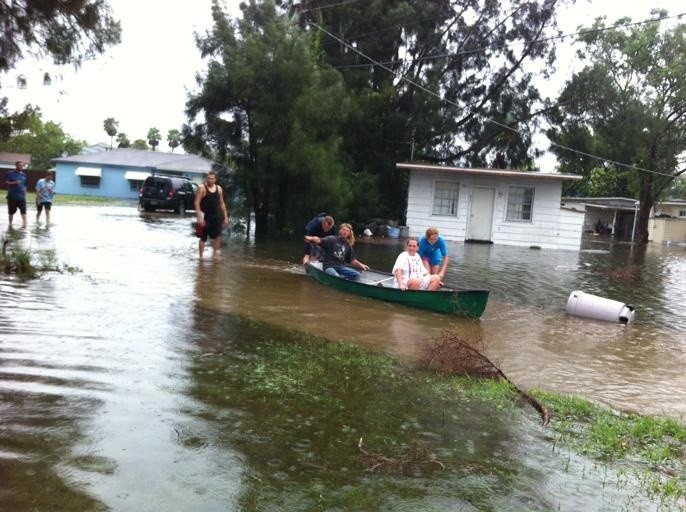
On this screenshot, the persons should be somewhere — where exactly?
[303,223,370,281]
[391,239,440,291]
[416,227,448,288]
[4,161,31,229]
[194,173,228,259]
[302,216,337,265]
[34,171,56,224]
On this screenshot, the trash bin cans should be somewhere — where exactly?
[566,290,636,325]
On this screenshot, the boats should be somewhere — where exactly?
[303,258,491,321]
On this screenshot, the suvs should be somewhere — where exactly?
[138,175,203,214]
